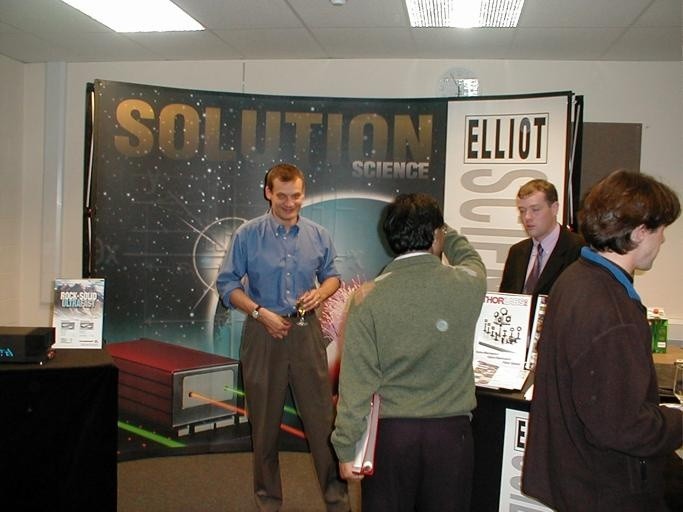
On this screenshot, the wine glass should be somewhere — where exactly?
[295,293,308,327]
[674,364,683,411]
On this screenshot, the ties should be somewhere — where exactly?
[521,243,543,294]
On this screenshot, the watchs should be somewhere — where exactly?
[251,305,261,320]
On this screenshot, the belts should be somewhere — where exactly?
[282,309,314,319]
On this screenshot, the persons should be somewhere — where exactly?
[216,163,351,512]
[521,170,683,512]
[499,180,588,361]
[330,192,486,512]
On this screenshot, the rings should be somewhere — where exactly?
[315,301,320,308]
